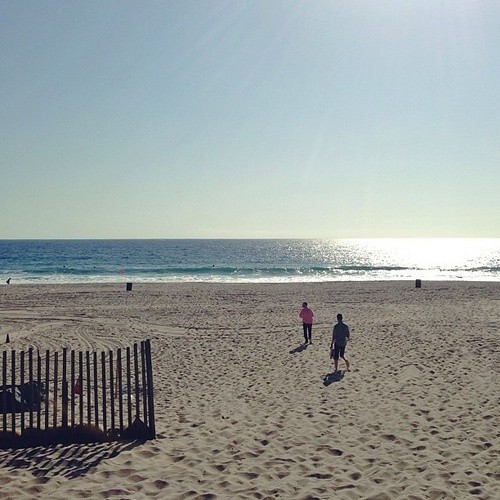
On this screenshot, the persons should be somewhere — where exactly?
[330,313,351,372]
[299,302,314,344]
